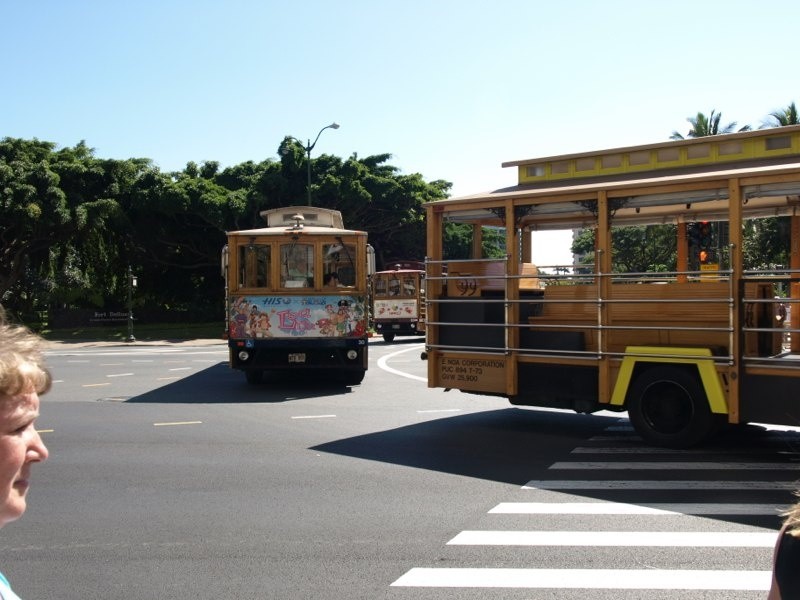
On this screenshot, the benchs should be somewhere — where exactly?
[445,261,786,359]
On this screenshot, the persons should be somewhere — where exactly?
[0,303,51,600]
[767,491,800,600]
[324,272,345,289]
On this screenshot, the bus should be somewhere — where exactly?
[221,206,370,385]
[420,124,800,449]
[369,257,446,343]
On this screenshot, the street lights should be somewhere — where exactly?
[281,122,340,207]
